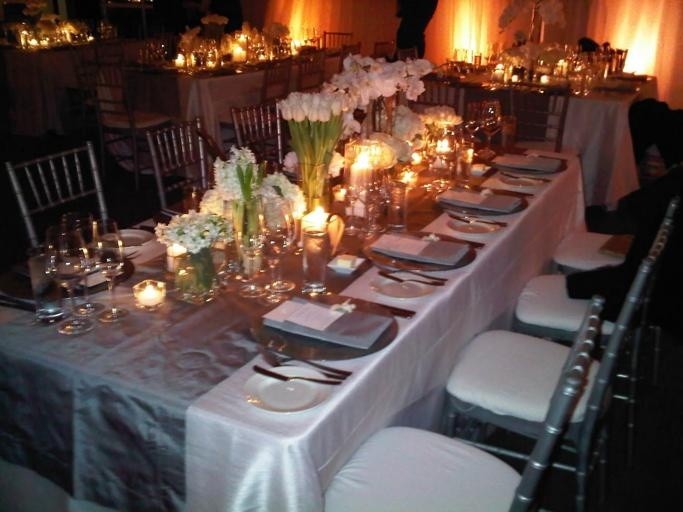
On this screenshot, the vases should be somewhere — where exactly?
[232,197,264,284]
[168,253,227,304]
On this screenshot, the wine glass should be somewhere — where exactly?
[342,95,503,241]
[233,195,296,305]
[45,210,129,334]
[8,20,113,51]
[171,27,324,79]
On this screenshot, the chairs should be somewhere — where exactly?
[553,196,676,376]
[514,228,669,470]
[5,141,110,253]
[324,293,605,511]
[442,273,656,510]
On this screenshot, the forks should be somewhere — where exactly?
[499,171,552,183]
[377,266,445,289]
[249,364,344,387]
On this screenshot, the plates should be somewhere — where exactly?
[244,365,330,413]
[498,176,544,187]
[100,228,153,249]
[368,272,436,301]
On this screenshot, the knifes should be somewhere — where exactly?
[263,346,353,376]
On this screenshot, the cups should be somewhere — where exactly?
[133,280,167,311]
[474,49,627,95]
[26,254,62,325]
[302,227,327,291]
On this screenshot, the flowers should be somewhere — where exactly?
[153,211,235,306]
[214,143,264,236]
